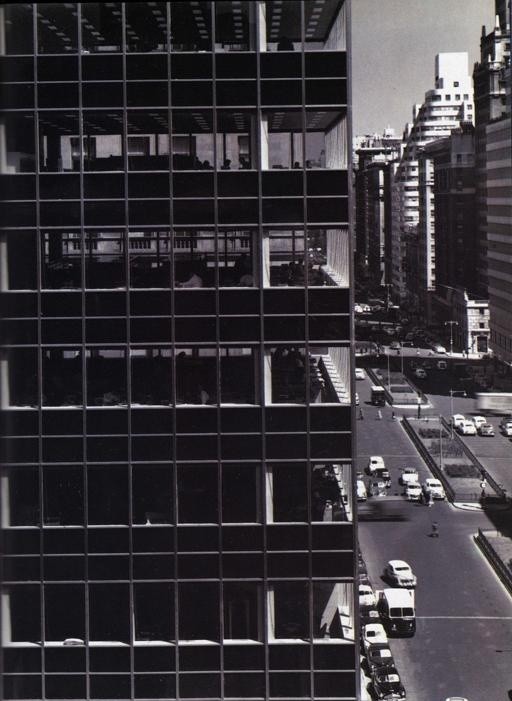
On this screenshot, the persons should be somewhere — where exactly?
[429,521,440,538]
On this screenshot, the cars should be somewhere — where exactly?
[355,392,359,406]
[410,359,448,379]
[355,542,416,701]
[355,368,365,380]
[452,414,512,437]
[356,454,445,503]
[354,300,446,355]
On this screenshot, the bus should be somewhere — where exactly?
[370,385,385,407]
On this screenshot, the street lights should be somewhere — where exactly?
[400,339,414,378]
[376,346,391,392]
[380,284,394,311]
[424,413,444,471]
[444,321,459,356]
[449,390,466,441]
